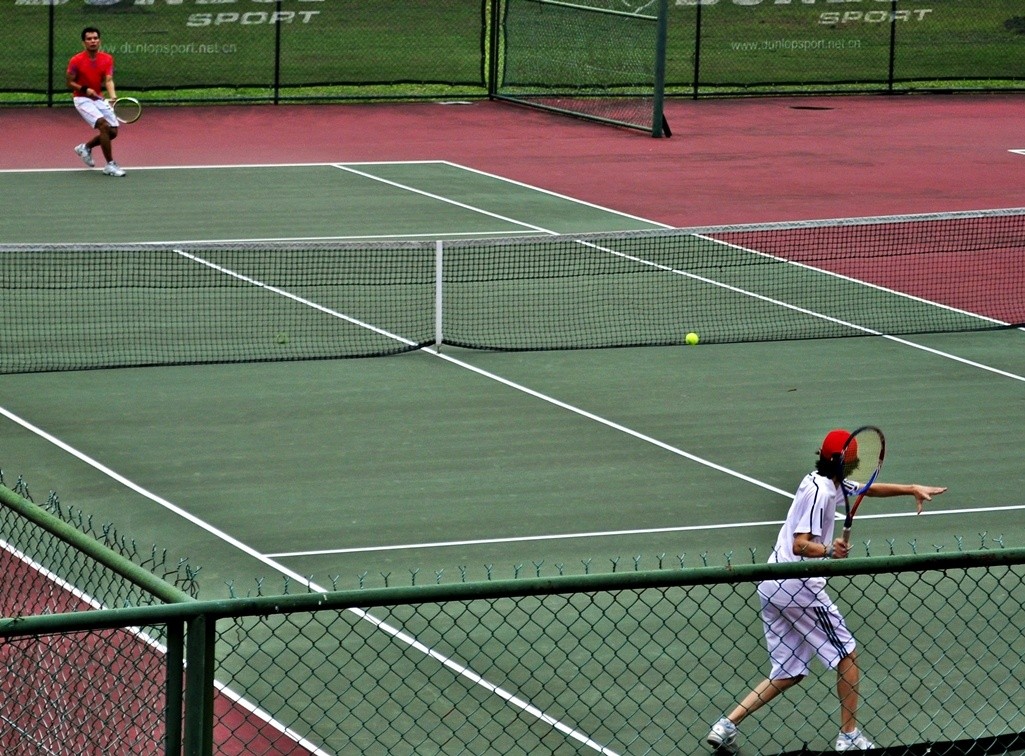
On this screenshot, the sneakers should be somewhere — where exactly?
[706,718,740,756]
[103,161,126,177]
[74,143,96,167]
[835,727,876,751]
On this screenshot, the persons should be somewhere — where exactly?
[708,428,947,750]
[66,26,127,178]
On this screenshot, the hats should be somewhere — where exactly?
[819,430,858,462]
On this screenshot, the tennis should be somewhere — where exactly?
[685,332,700,346]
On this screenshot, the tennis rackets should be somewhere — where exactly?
[834,424,886,548]
[91,94,143,124]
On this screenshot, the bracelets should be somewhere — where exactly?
[80,85,87,94]
[829,545,833,556]
